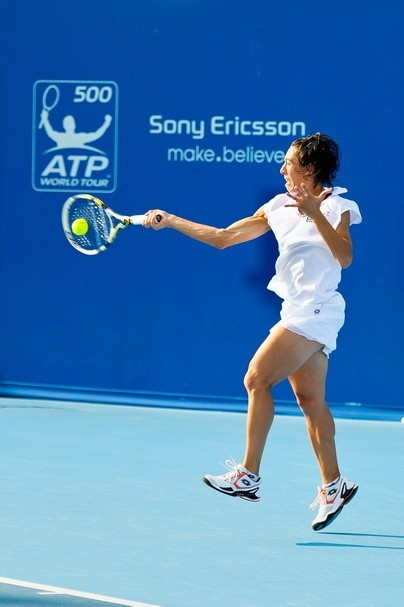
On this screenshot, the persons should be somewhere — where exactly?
[144,130,364,530]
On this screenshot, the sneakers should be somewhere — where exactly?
[203,470,262,503]
[311,478,357,531]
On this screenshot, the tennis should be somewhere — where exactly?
[71,218,88,235]
